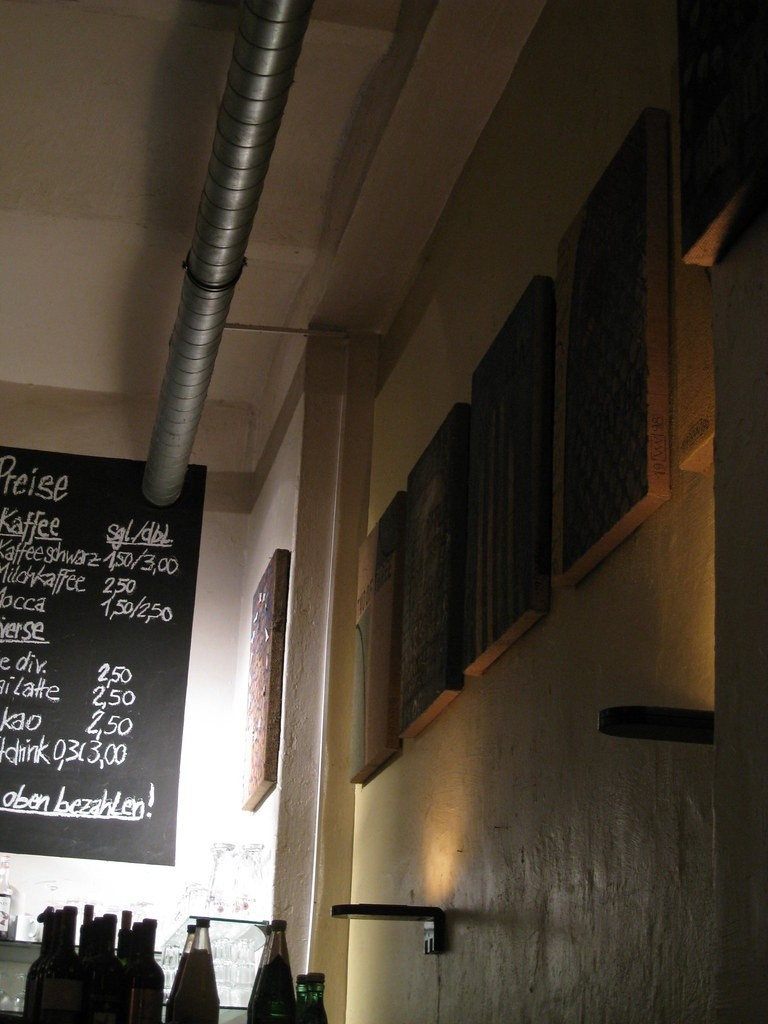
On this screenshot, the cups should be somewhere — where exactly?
[166,937,261,1009]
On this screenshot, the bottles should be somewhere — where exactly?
[247,920,294,1024]
[165,918,219,1024]
[179,843,272,937]
[0,862,17,941]
[24,904,164,1024]
[295,972,327,1024]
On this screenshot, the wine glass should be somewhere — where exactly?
[14,973,24,1008]
[0,972,11,1009]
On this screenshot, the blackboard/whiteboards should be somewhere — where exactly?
[0,444,210,866]
